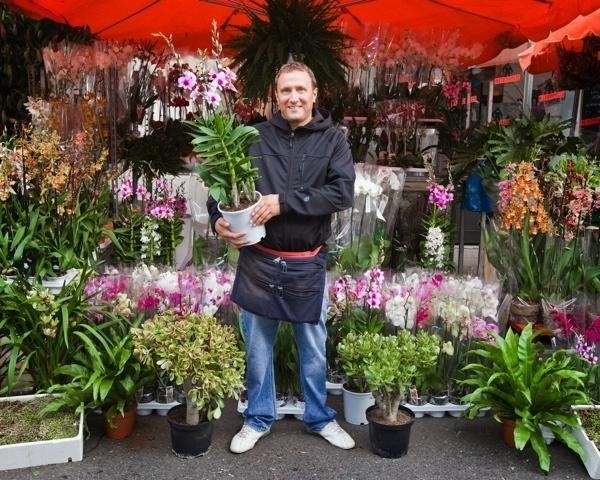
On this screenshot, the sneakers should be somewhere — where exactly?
[229,421,271,454]
[305,418,355,450]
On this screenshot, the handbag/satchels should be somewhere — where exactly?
[230,245,326,325]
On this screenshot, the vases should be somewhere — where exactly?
[400,396,492,419]
[135,400,180,417]
[541,304,576,326]
[2,267,82,303]
[509,303,540,325]
[237,395,307,420]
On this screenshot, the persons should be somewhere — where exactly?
[206,61,356,454]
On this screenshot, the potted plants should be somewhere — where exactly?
[128,309,245,459]
[327,308,400,426]
[34,311,158,440]
[178,110,267,247]
[450,322,600,472]
[336,329,441,459]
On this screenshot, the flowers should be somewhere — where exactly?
[536,151,600,302]
[149,18,240,128]
[418,174,457,269]
[0,121,110,276]
[481,160,577,303]
[112,175,186,272]
[83,264,499,404]
